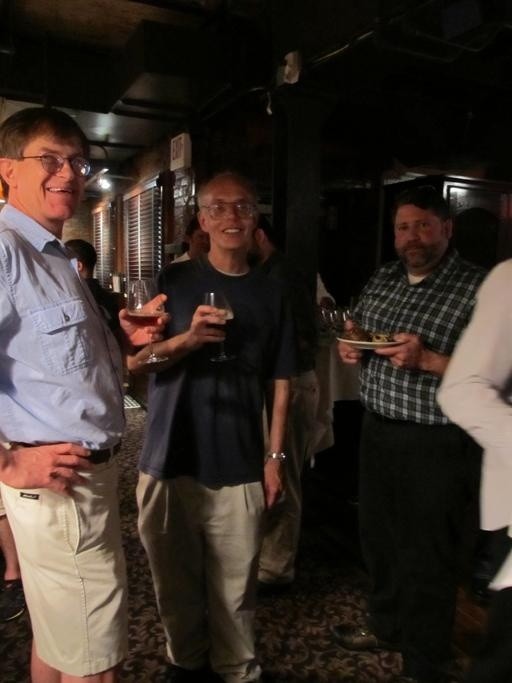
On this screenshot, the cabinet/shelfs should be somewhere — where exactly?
[379,172,512,265]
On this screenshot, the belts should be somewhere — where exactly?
[10,438,122,466]
[369,410,422,426]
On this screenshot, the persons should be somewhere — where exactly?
[1,106,171,682]
[121,172,511,683]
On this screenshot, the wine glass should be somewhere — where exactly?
[128,277,170,365]
[200,291,240,362]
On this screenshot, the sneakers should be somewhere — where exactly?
[0,571,28,623]
[166,661,213,683]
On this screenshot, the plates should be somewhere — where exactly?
[334,336,407,351]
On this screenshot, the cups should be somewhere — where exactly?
[322,304,351,329]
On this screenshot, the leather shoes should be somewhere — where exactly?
[328,620,401,652]
[386,669,449,683]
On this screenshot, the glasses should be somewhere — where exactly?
[14,150,93,177]
[198,200,258,217]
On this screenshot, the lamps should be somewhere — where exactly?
[96,174,138,191]
